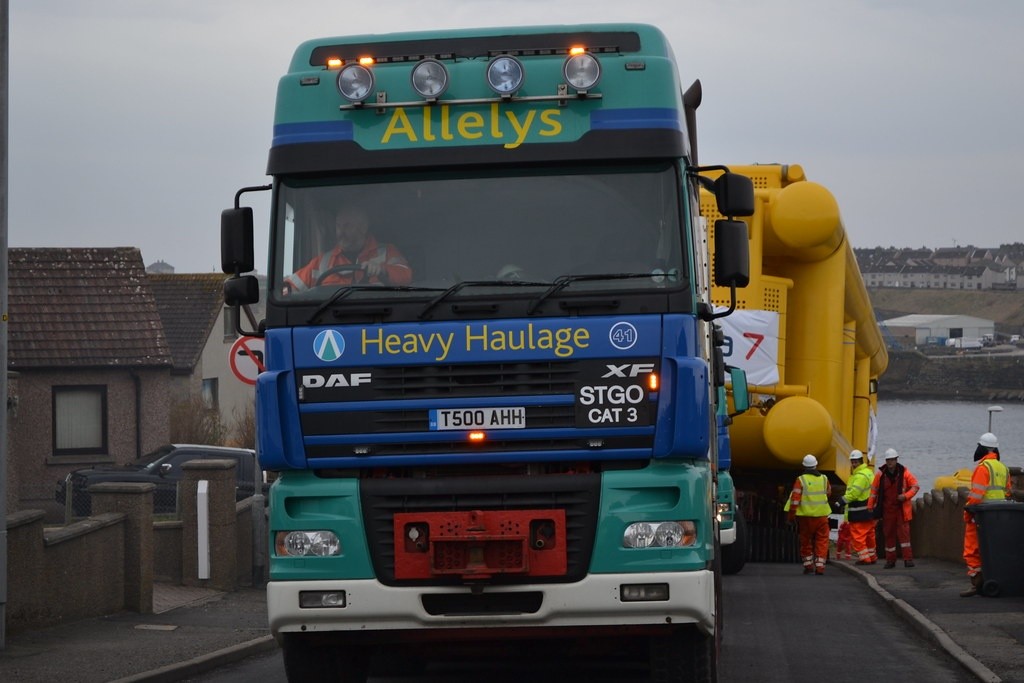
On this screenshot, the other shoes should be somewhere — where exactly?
[803,568,814,574]
[815,567,824,575]
[855,560,877,565]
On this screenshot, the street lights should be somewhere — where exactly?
[987,406,1004,433]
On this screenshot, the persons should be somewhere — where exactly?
[959,433,1013,598]
[283,207,413,293]
[837,520,852,560]
[835,450,879,564]
[783,454,832,575]
[867,448,920,569]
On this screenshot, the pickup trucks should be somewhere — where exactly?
[55,443,274,513]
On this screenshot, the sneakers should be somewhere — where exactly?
[884,562,895,569]
[960,585,981,597]
[904,560,916,568]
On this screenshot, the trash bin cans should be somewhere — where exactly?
[963,502,1024,597]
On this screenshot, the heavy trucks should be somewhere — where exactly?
[221,24,755,683]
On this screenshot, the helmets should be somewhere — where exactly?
[802,454,818,467]
[885,448,899,459]
[848,449,863,459]
[977,432,999,448]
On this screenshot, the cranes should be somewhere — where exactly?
[876,311,902,350]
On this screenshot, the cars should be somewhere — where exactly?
[1010,335,1021,344]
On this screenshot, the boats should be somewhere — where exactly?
[934,469,973,492]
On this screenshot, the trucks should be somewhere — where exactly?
[946,337,983,350]
